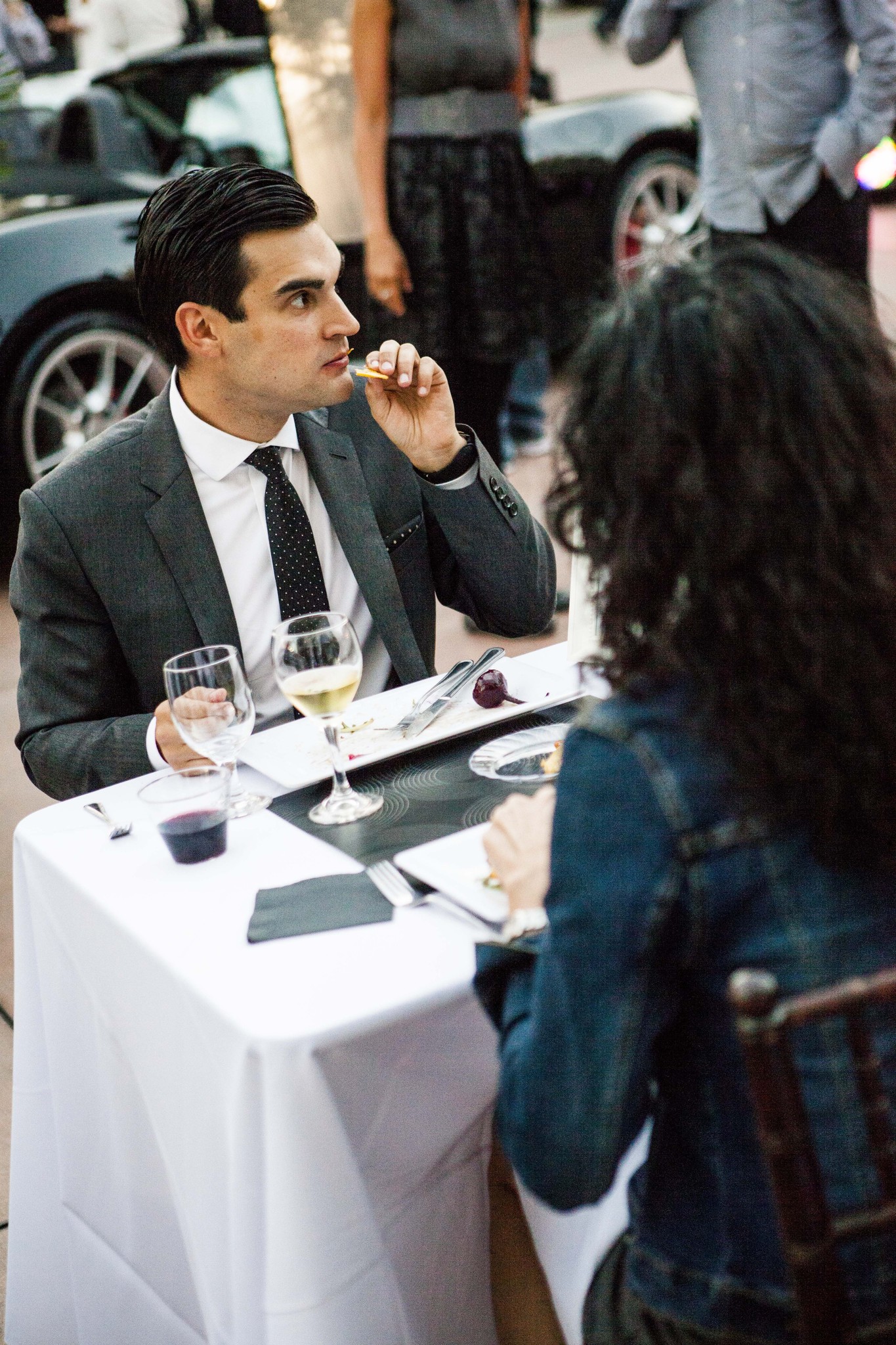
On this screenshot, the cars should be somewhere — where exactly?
[0,36,700,580]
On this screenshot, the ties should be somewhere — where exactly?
[247,447,341,721]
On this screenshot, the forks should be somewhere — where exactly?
[366,860,503,940]
[373,660,474,731]
[83,803,131,839]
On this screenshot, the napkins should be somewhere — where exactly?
[248,866,401,944]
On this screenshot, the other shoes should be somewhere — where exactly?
[516,428,550,458]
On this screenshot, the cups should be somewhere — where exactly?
[137,766,230,865]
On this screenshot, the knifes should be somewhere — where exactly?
[405,647,506,741]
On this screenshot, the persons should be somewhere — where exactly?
[499,311,552,462]
[351,0,527,478]
[10,161,557,802]
[615,0,896,281]
[0,0,189,109]
[470,245,896,1345]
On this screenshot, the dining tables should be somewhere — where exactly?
[5,641,626,1345]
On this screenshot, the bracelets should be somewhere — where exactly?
[414,431,476,484]
[501,908,546,943]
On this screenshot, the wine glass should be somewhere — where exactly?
[163,644,273,819]
[271,611,384,825]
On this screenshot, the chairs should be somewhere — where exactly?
[727,965,896,1345]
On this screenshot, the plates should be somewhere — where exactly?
[469,723,574,783]
[393,820,510,922]
[237,656,586,789]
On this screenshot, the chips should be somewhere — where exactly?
[355,368,389,380]
